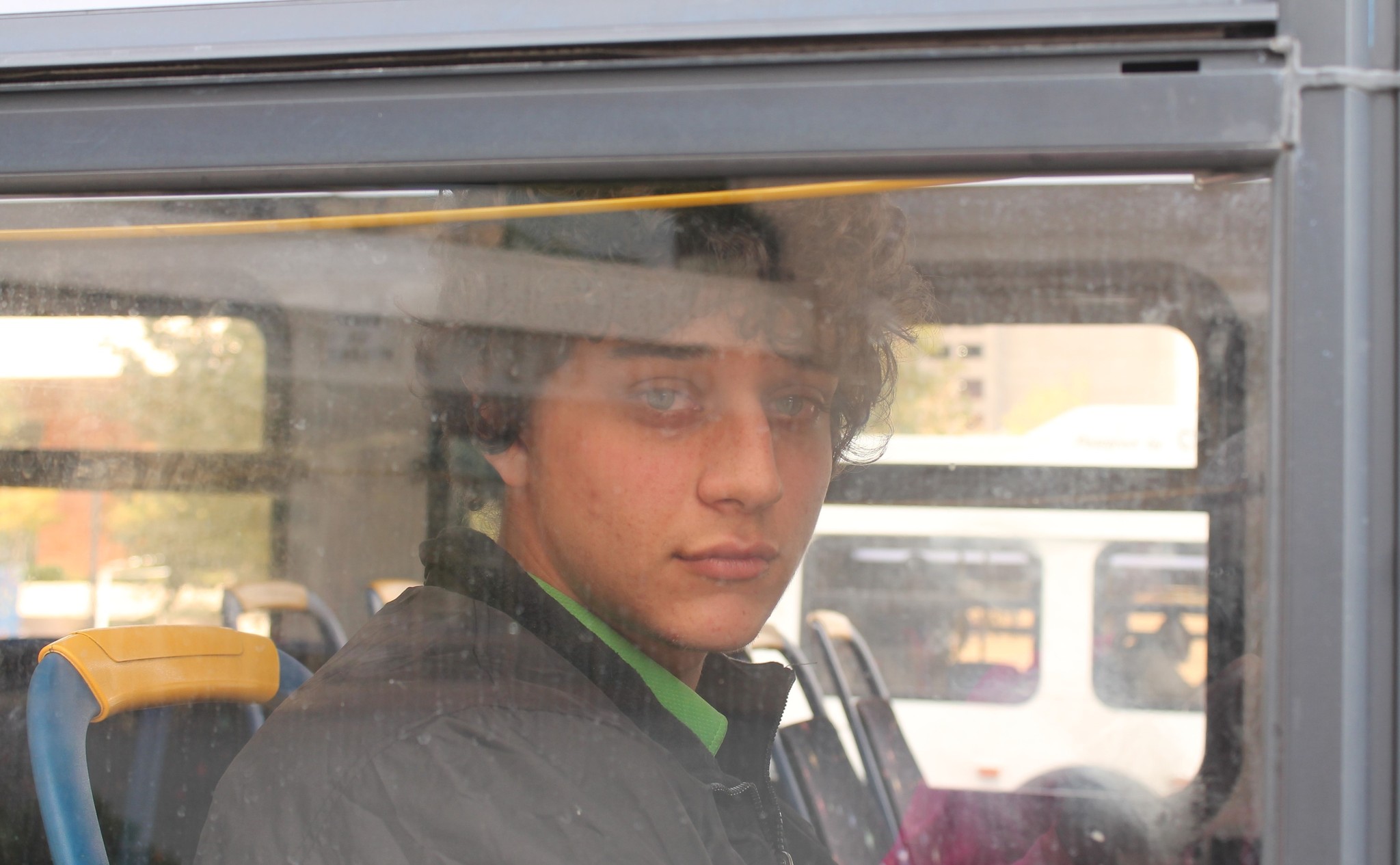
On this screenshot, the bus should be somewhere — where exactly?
[2,0,1400,865]
[5,376,1199,808]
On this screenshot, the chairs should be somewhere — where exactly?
[26,577,923,865]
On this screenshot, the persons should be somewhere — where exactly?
[198,179,931,865]
[1126,619,1202,713]
